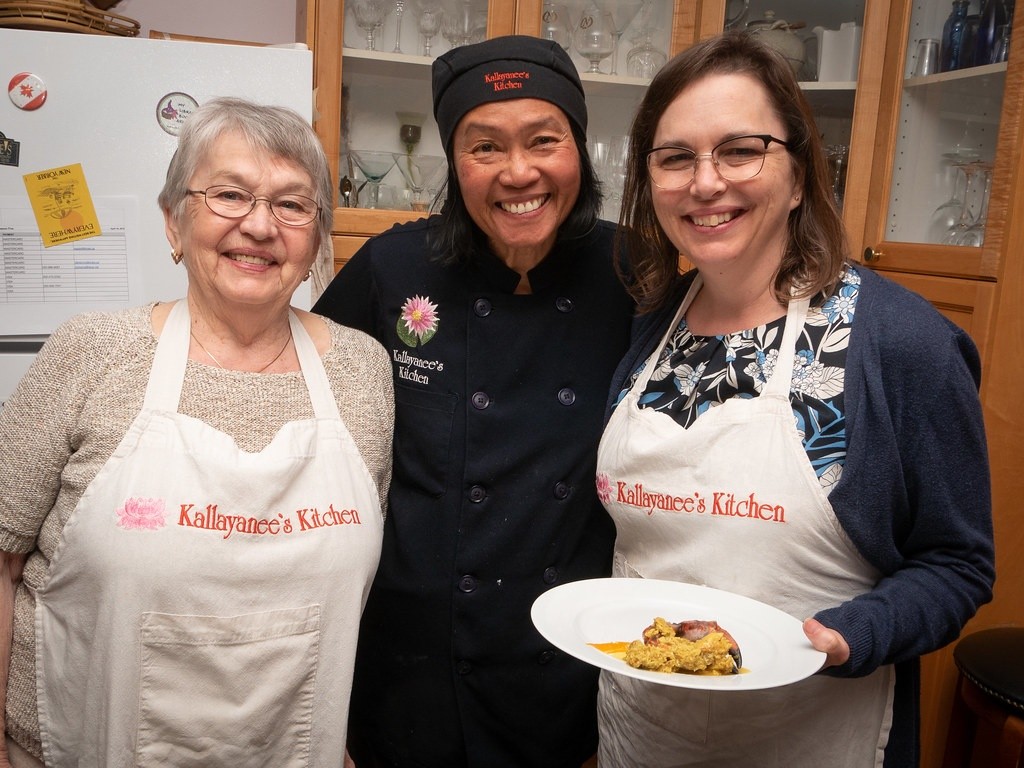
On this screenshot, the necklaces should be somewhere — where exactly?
[191,331,291,373]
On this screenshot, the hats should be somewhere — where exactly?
[431,34,588,154]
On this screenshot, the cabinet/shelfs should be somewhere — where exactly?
[294,0,1024,768]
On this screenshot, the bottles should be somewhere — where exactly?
[939,0,1017,72]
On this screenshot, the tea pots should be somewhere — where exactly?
[727,10,817,80]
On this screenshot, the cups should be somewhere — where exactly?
[913,38,939,76]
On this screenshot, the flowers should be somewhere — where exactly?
[395,293,439,347]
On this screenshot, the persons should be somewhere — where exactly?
[308,35,676,768]
[0,98,395,768]
[596,33,995,767]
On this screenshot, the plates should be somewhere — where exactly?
[531,577,827,690]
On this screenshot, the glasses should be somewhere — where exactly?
[641,133,791,190]
[186,186,323,228]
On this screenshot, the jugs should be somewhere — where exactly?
[811,21,863,83]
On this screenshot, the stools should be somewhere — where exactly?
[941,628,1024,768]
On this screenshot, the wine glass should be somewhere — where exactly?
[345,0,488,57]
[338,112,447,211]
[926,152,996,247]
[541,3,666,80]
[588,135,638,226]
